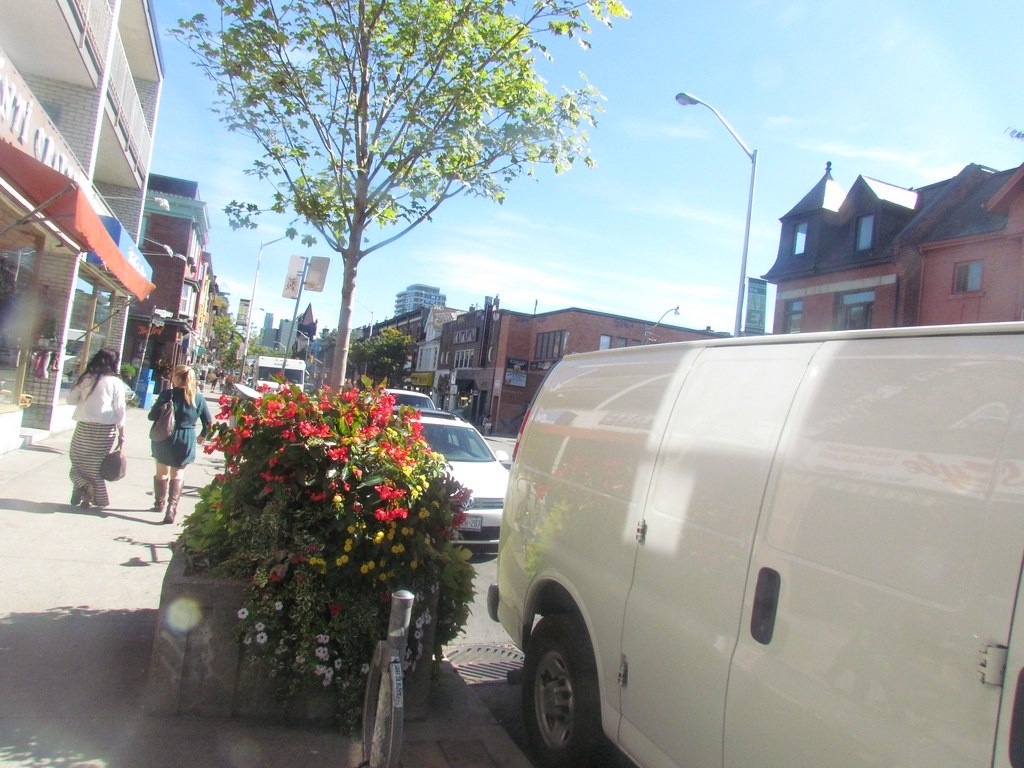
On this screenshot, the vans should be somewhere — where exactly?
[482,328,1024,768]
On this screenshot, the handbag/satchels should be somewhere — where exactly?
[101,444,127,482]
[149,389,175,441]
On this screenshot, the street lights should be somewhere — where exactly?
[294,328,310,364]
[238,234,317,382]
[676,92,758,338]
[273,340,286,354]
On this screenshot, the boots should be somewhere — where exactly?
[153,476,168,512]
[164,478,184,523]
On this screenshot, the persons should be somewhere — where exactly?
[146,364,212,523]
[210,369,226,395]
[68,346,127,511]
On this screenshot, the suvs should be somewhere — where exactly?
[384,410,514,546]
[366,389,440,416]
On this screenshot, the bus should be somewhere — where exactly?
[253,356,310,400]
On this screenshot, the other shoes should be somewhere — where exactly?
[81,502,90,510]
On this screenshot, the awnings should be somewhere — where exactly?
[0,139,156,302]
